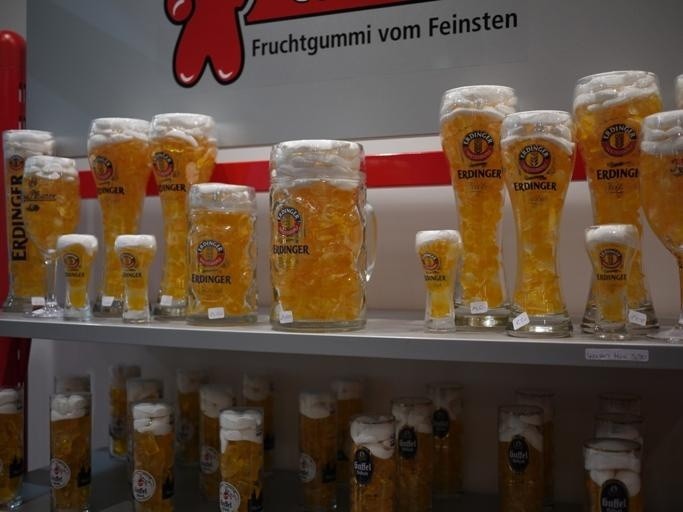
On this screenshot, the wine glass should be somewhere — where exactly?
[636,108,682,345]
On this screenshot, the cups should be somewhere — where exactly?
[412,228,465,335]
[87,116,158,325]
[183,181,261,327]
[496,107,577,338]
[0,362,647,512]
[583,224,641,341]
[438,82,523,328]
[267,134,383,332]
[1,123,100,323]
[569,69,661,336]
[148,112,217,318]
